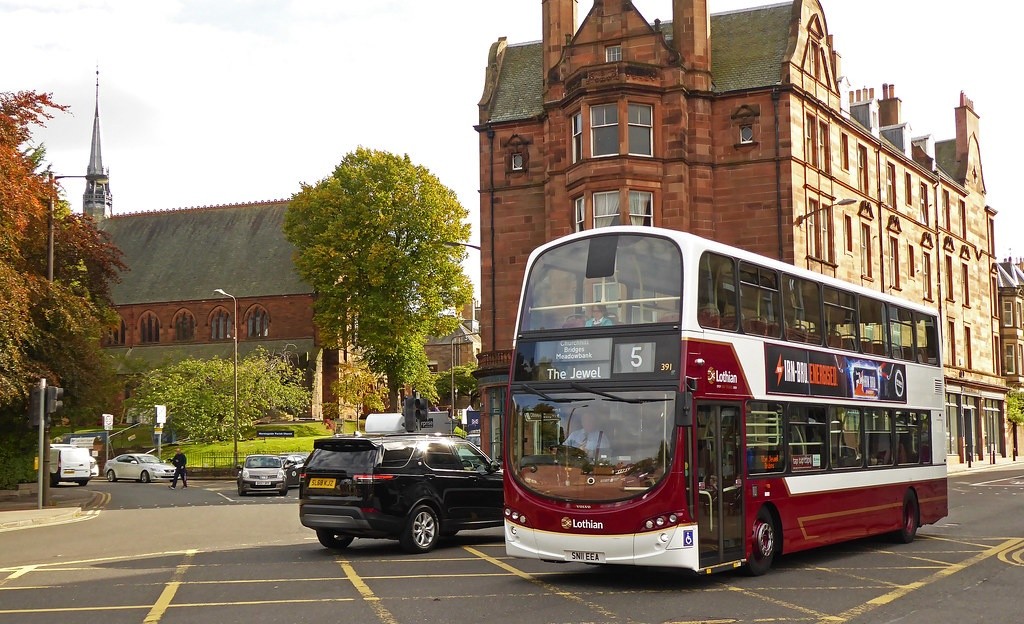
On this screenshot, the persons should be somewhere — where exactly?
[561,412,611,462]
[584,300,612,327]
[168,447,188,490]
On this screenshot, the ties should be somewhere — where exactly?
[580,432,589,450]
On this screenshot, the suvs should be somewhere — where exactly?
[298,430,503,555]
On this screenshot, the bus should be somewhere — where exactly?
[503,225,948,576]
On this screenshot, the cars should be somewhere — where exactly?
[236,454,290,496]
[103,452,181,483]
[278,454,309,486]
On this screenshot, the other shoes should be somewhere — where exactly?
[168,485,175,490]
[182,486,188,490]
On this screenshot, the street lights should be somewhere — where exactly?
[214,288,238,466]
[47,172,109,283]
[450,331,481,418]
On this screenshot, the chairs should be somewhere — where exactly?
[839,445,934,467]
[652,304,930,364]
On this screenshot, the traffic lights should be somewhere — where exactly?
[401,398,417,433]
[46,386,64,414]
[415,398,428,421]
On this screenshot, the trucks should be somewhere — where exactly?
[34,443,99,488]
